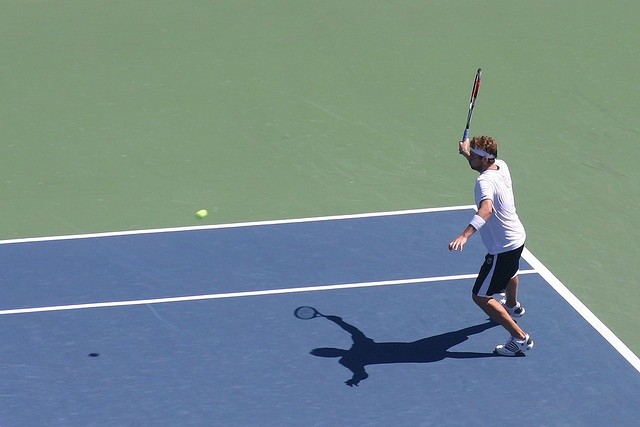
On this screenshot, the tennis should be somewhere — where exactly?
[196,209,208,221]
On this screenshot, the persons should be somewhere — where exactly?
[448,135,535,357]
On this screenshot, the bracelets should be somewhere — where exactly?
[468,214,485,231]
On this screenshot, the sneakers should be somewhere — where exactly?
[498,299,525,318]
[495,333,534,356]
